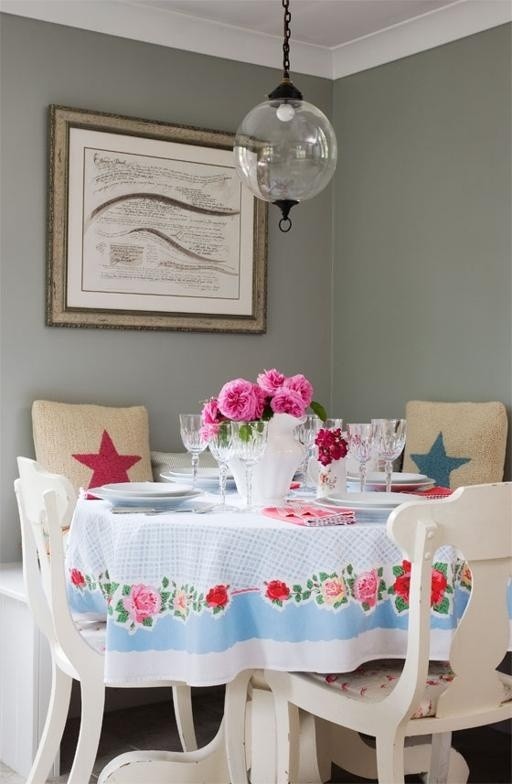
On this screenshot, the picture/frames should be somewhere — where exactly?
[44,103,270,335]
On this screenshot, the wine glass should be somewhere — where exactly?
[178,409,410,514]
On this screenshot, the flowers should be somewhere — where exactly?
[314,428,349,467]
[198,369,327,449]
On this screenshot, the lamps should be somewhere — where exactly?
[232,0,339,234]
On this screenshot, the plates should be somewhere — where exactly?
[343,469,435,493]
[159,466,245,490]
[308,492,428,518]
[85,480,205,510]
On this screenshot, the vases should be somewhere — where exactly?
[230,413,309,507]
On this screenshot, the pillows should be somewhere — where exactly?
[401,401,509,490]
[30,399,156,532]
